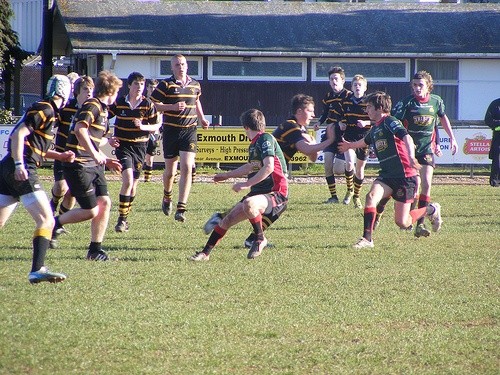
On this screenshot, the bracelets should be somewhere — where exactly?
[15,163,22,165]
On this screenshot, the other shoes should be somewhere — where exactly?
[400,224,413,232]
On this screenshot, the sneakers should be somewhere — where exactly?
[175,212,185,222]
[352,195,362,210]
[189,250,209,261]
[414,223,430,238]
[373,211,383,230]
[28,266,65,285]
[50,227,71,249]
[352,237,374,249]
[87,249,118,261]
[328,197,338,203]
[204,213,222,235]
[247,238,267,259]
[124,221,129,231]
[114,220,125,233]
[162,197,172,216]
[343,191,354,204]
[245,233,256,248]
[428,202,443,232]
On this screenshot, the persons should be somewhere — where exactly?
[50,72,122,261]
[151,53,209,222]
[314,66,355,204]
[0,74,75,283]
[338,92,441,247]
[484,97,500,187]
[374,71,458,237]
[204,96,336,248]
[106,72,163,232]
[339,74,372,209]
[190,108,288,260]
